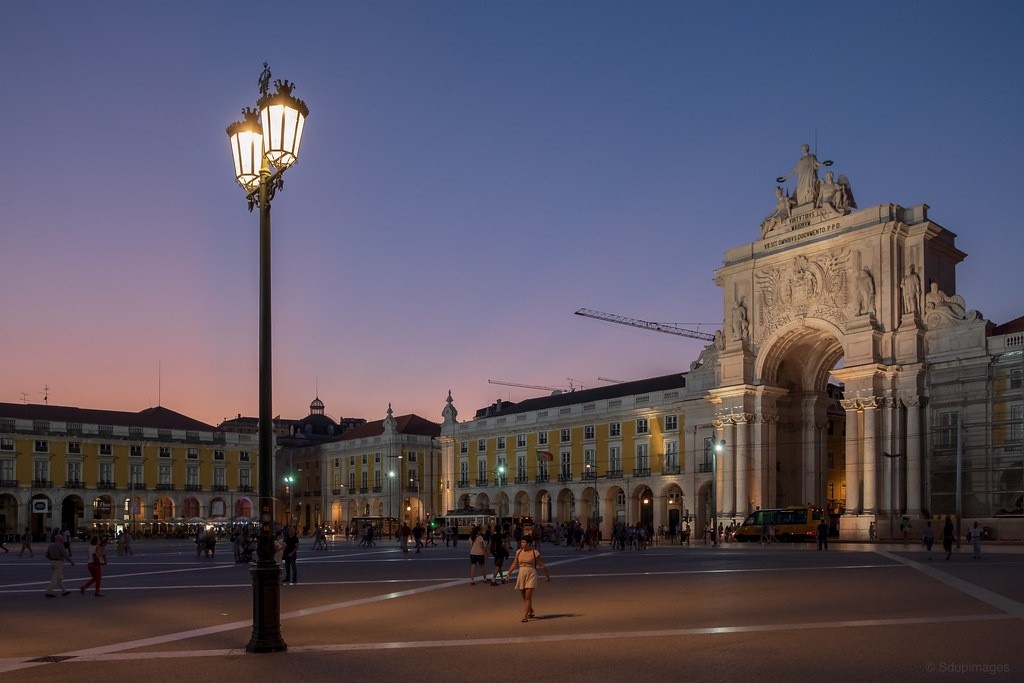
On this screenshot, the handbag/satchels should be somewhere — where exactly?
[92,546,106,565]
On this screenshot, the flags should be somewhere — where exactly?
[536,451,553,461]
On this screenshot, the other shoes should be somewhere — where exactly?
[471,582,477,585]
[46,594,57,598]
[95,593,106,596]
[81,587,84,595]
[502,579,506,584]
[483,579,490,583]
[291,579,297,583]
[62,591,72,596]
[282,578,289,582]
[490,581,499,586]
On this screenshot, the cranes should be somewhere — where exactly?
[573,307,716,342]
[488,376,582,393]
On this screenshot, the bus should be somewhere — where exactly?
[431,513,497,539]
[732,502,826,544]
[350,516,400,537]
[497,515,534,539]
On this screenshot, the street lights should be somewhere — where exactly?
[389,438,403,541]
[410,478,420,525]
[585,464,599,542]
[497,465,506,536]
[284,475,294,536]
[132,471,141,540]
[340,484,349,542]
[224,60,311,655]
[712,444,723,548]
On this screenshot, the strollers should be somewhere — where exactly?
[237,540,256,563]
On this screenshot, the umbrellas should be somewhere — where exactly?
[94,515,259,533]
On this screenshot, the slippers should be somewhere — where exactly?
[522,619,528,622]
[527,614,535,619]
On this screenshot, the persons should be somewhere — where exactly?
[533,516,741,552]
[166,522,299,583]
[505,535,551,622]
[19,527,33,557]
[758,523,780,544]
[81,536,106,597]
[855,269,874,316]
[729,300,748,342]
[760,144,846,240]
[96,524,145,556]
[903,517,909,545]
[468,519,525,586]
[815,518,837,550]
[398,521,459,553]
[45,535,74,597]
[51,526,72,557]
[0,523,9,553]
[310,523,376,550]
[692,330,724,364]
[920,516,984,560]
[899,267,966,317]
[869,521,875,540]
